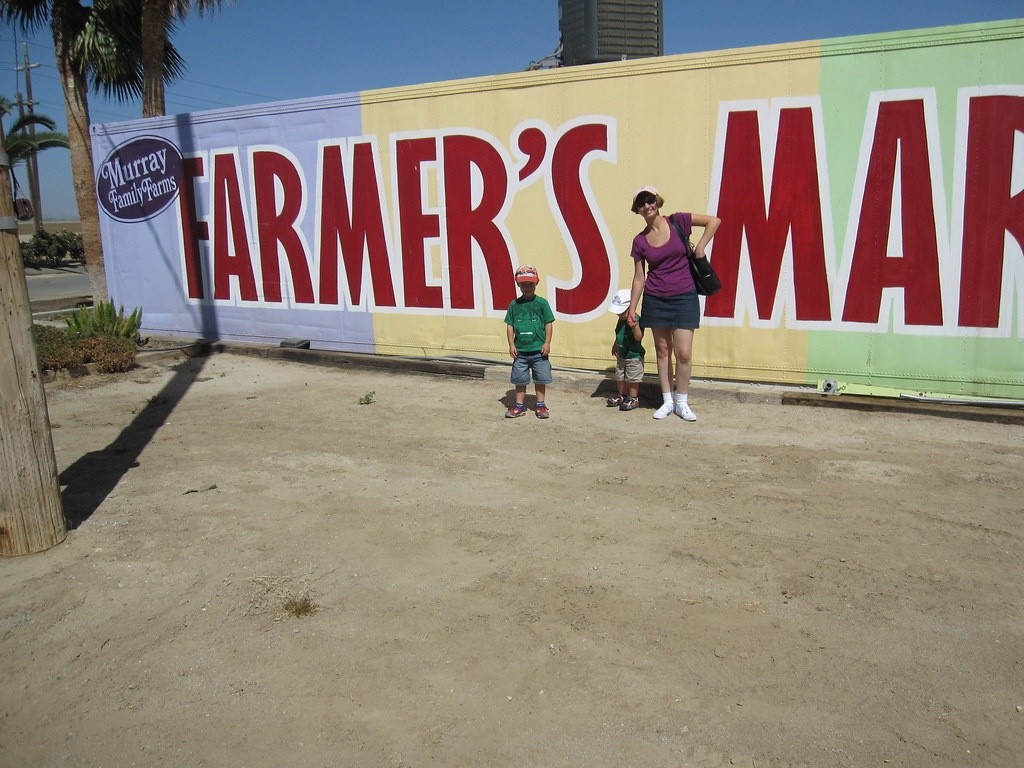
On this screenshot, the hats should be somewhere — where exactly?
[608,288,632,315]
[633,185,657,203]
[515,264,539,284]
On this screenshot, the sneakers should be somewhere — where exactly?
[607,394,626,406]
[505,405,526,418]
[676,404,697,421]
[619,396,639,411]
[536,404,549,419]
[653,400,676,419]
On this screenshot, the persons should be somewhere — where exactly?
[504,264,555,420]
[606,288,646,411]
[626,186,721,422]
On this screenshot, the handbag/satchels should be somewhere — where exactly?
[669,213,721,295]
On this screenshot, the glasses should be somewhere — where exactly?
[638,198,656,208]
